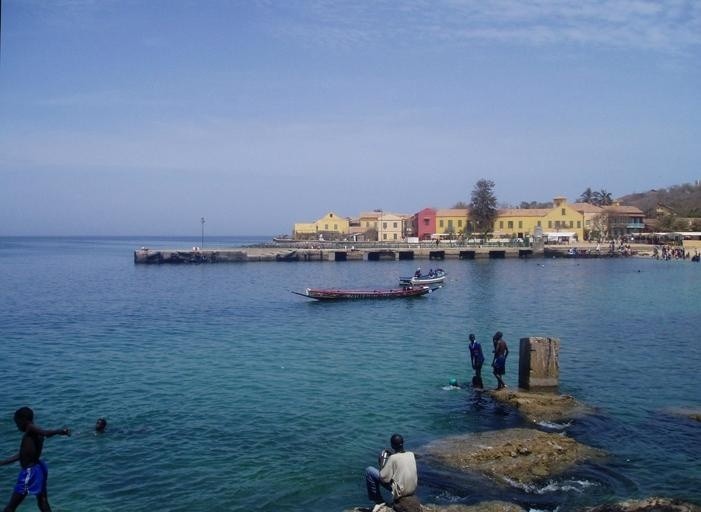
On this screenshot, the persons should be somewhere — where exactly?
[468,334,485,390]
[426,268,437,276]
[492,331,509,389]
[492,334,497,367]
[566,237,687,261]
[95,418,108,433]
[365,434,418,511]
[0,407,70,512]
[415,267,423,277]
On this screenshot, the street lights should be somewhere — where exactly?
[199,215,205,249]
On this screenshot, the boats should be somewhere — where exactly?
[397,269,447,285]
[285,283,441,301]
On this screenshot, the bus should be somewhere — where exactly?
[628,229,700,247]
[429,233,493,244]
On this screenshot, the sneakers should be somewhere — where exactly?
[372,502,386,512]
